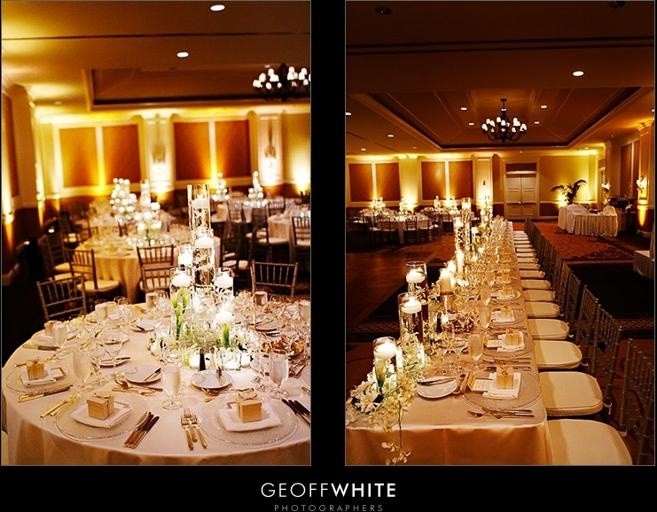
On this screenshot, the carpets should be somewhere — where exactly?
[347,220,653,467]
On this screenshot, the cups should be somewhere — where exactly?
[372,336,396,388]
[397,291,422,342]
[405,261,429,324]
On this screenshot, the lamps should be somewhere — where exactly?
[252,63,310,103]
[600,180,612,193]
[636,175,648,193]
[479,97,526,145]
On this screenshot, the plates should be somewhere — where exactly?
[415,376,457,398]
[5,304,311,447]
[464,222,542,410]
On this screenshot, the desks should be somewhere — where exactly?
[558,204,619,237]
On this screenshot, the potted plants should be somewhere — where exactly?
[170,289,193,347]
[217,323,240,372]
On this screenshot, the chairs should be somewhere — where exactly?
[219,236,239,295]
[380,217,396,244]
[139,266,172,293]
[292,216,311,280]
[66,248,119,309]
[251,260,298,301]
[250,207,288,264]
[436,213,444,237]
[441,211,452,232]
[529,285,654,465]
[363,218,378,240]
[220,225,257,289]
[472,209,480,225]
[268,201,285,219]
[419,215,434,239]
[632,223,654,279]
[37,235,84,284]
[137,245,175,290]
[240,205,267,257]
[352,216,362,237]
[73,227,97,244]
[36,273,88,321]
[58,210,82,247]
[404,216,418,241]
[513,218,570,320]
[45,219,72,272]
[221,220,241,260]
[227,207,266,258]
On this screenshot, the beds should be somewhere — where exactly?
[0,302,312,466]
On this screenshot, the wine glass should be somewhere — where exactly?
[429,216,507,375]
[53,290,311,406]
